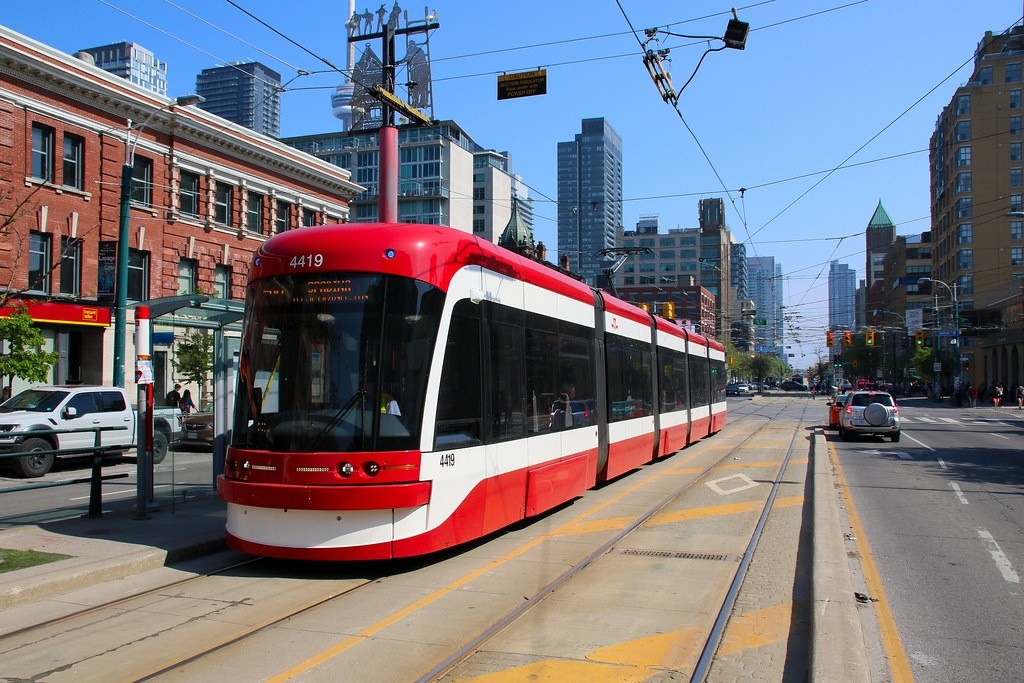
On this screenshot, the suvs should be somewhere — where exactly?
[780,381,807,391]
[836,390,901,442]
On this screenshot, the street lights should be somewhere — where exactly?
[113,94,206,386]
[916,277,963,382]
[873,309,909,391]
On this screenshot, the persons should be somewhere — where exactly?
[990,382,1004,410]
[959,382,975,403]
[166,384,181,407]
[547,380,588,432]
[331,363,401,416]
[179,389,198,427]
[1015,384,1024,410]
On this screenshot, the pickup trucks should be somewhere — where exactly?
[0,384,189,479]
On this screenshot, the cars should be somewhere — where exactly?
[839,378,925,398]
[180,402,216,452]
[725,382,770,397]
[826,394,848,431]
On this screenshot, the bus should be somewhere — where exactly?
[216,221,727,562]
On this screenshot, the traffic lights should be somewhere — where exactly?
[834,355,838,367]
[916,331,924,347]
[844,331,852,346]
[838,354,842,367]
[866,329,875,346]
[827,333,834,347]
